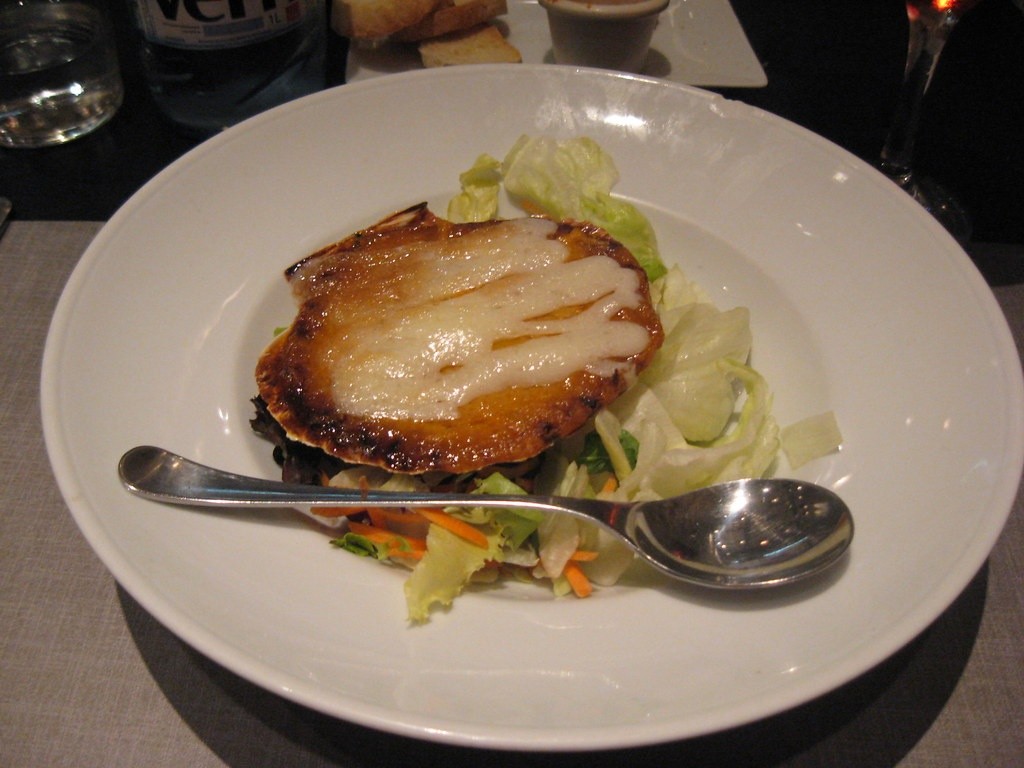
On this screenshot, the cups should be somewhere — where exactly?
[0,0,127,149]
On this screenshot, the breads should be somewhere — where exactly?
[338,0,523,69]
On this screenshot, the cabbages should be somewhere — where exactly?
[309,131,848,626]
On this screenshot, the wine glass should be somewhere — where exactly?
[872,2,973,256]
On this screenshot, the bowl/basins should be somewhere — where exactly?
[538,0,670,74]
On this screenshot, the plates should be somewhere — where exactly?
[345,0,772,94]
[38,63,1024,753]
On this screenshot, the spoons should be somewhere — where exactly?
[117,443,856,593]
